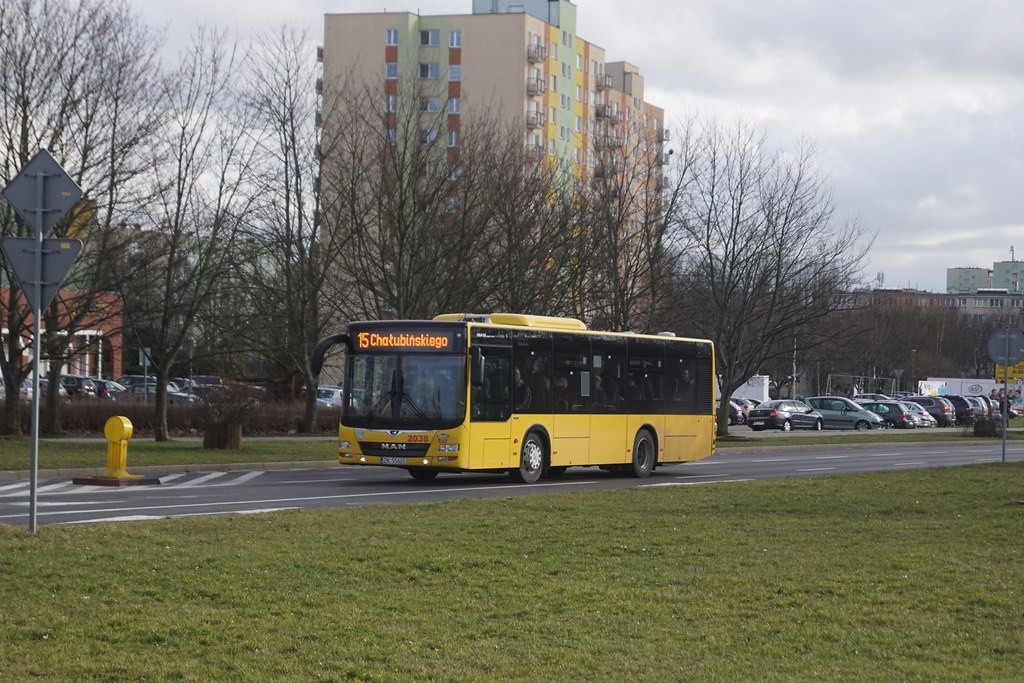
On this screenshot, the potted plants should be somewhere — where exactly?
[195,387,255,447]
[973,407,1006,438]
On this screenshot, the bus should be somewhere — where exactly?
[310,312,718,486]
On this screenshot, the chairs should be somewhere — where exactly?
[825,402,833,411]
[525,376,702,413]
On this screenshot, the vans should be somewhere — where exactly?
[804,396,886,432]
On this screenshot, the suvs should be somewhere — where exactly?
[899,396,957,427]
[938,394,977,425]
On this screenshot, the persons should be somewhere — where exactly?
[523,356,577,413]
[1007,389,1014,400]
[989,389,1000,400]
[876,386,884,394]
[997,388,1005,397]
[1013,389,1022,403]
[503,367,531,414]
[592,374,606,406]
[999,394,1011,427]
[678,368,694,400]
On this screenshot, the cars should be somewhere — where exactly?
[849,391,937,429]
[0,372,373,413]
[966,394,1018,423]
[730,397,755,424]
[748,398,763,408]
[715,398,742,426]
[747,399,825,433]
